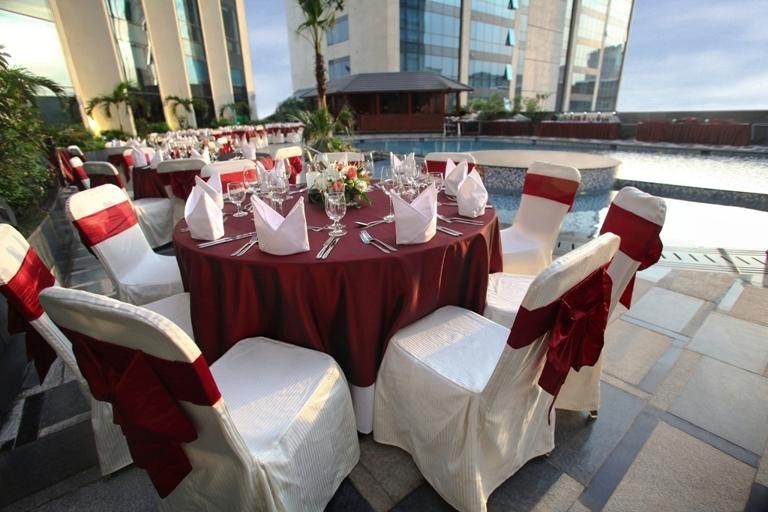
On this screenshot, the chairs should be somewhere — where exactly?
[368,230,625,512]
[47,119,493,219]
[0,219,196,484]
[494,159,583,286]
[62,182,186,303]
[35,285,363,512]
[483,184,668,425]
[79,159,176,251]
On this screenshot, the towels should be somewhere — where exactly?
[386,181,440,247]
[181,184,224,244]
[247,193,311,257]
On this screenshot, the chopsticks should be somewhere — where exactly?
[194,230,258,249]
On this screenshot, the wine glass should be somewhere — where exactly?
[324,192,349,237]
[324,176,347,230]
[226,152,443,221]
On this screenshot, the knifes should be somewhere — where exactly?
[444,202,492,209]
[322,235,339,261]
[316,235,331,260]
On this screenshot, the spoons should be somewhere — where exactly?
[360,214,397,229]
[353,220,393,225]
[446,195,457,201]
[436,213,485,226]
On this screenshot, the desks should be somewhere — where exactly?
[496,110,754,146]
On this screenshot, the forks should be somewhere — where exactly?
[361,228,398,253]
[229,235,257,257]
[357,231,391,258]
